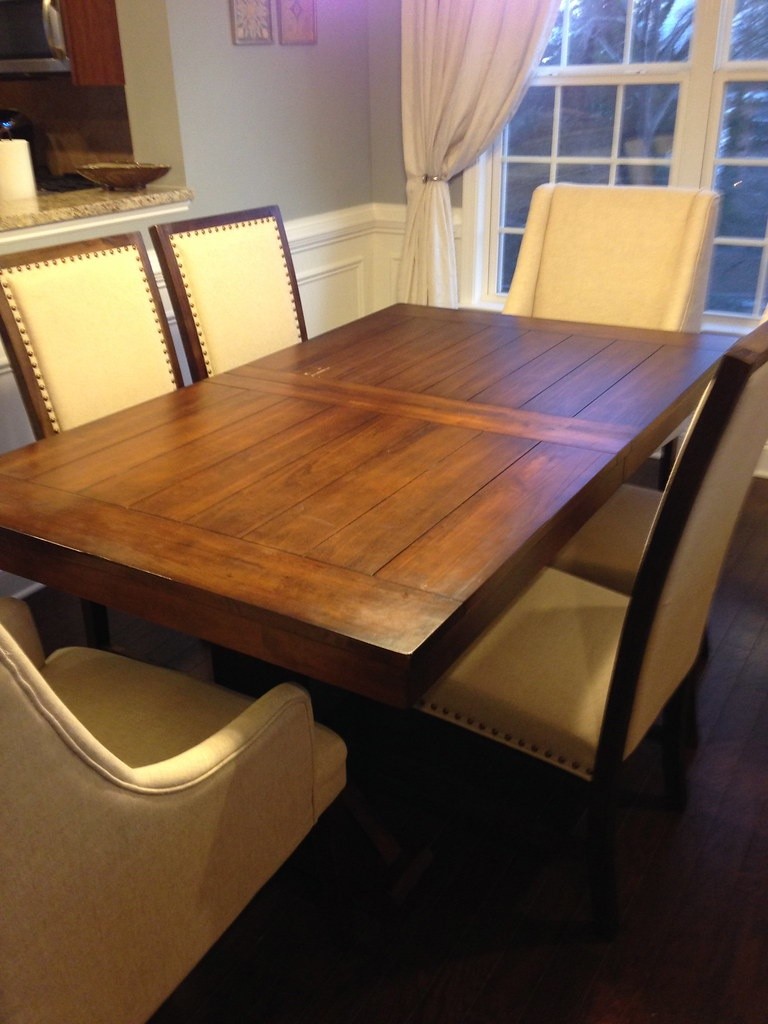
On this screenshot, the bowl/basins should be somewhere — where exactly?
[74,161,173,191]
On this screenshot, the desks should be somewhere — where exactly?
[0,302,743,708]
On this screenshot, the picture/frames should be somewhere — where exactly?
[229,0,274,45]
[276,0,318,47]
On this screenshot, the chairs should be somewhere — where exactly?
[149,203,310,384]
[621,132,653,187]
[0,231,184,648]
[502,180,720,491]
[554,484,714,733]
[0,593,349,1024]
[355,321,768,943]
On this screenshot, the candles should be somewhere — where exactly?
[0,126,36,202]
